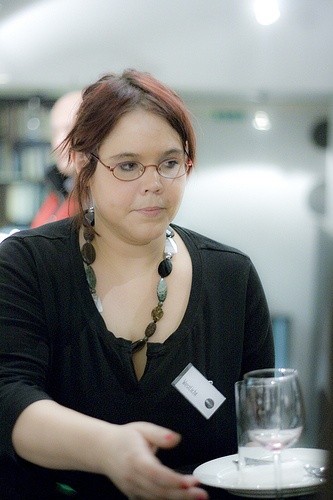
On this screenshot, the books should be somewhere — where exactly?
[0,101,52,227]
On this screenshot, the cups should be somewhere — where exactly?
[235,381,280,471]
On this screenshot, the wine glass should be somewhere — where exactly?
[242,368,305,500]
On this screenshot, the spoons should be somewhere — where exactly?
[302,463,329,477]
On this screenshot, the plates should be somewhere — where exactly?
[193,447,330,497]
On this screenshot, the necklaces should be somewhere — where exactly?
[82,205,179,355]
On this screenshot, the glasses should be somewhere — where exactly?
[89,149,196,183]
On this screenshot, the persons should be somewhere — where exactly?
[0,70,277,500]
[28,87,93,228]
